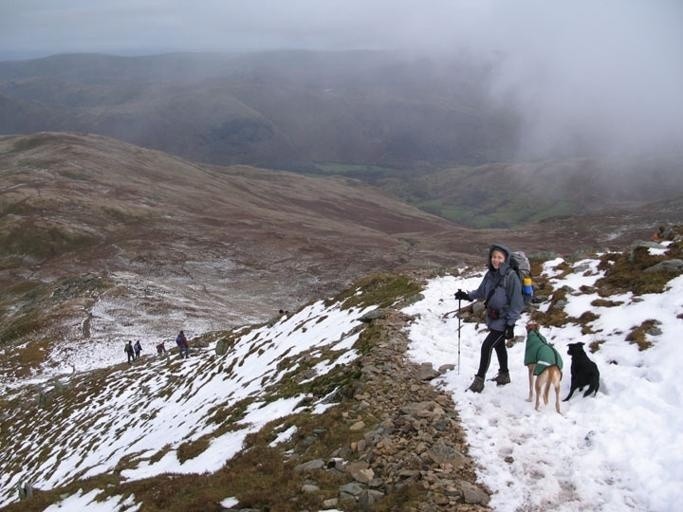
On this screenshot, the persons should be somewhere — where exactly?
[454,242,525,394]
[155,342,166,355]
[176,331,189,358]
[124,340,142,364]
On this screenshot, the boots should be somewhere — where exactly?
[466,376,485,393]
[488,369,510,385]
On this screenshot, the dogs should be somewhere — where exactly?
[524,323,561,413]
[562,342,599,401]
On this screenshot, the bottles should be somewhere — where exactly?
[522,276,533,294]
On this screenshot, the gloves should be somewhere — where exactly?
[455,291,473,302]
[504,325,515,340]
